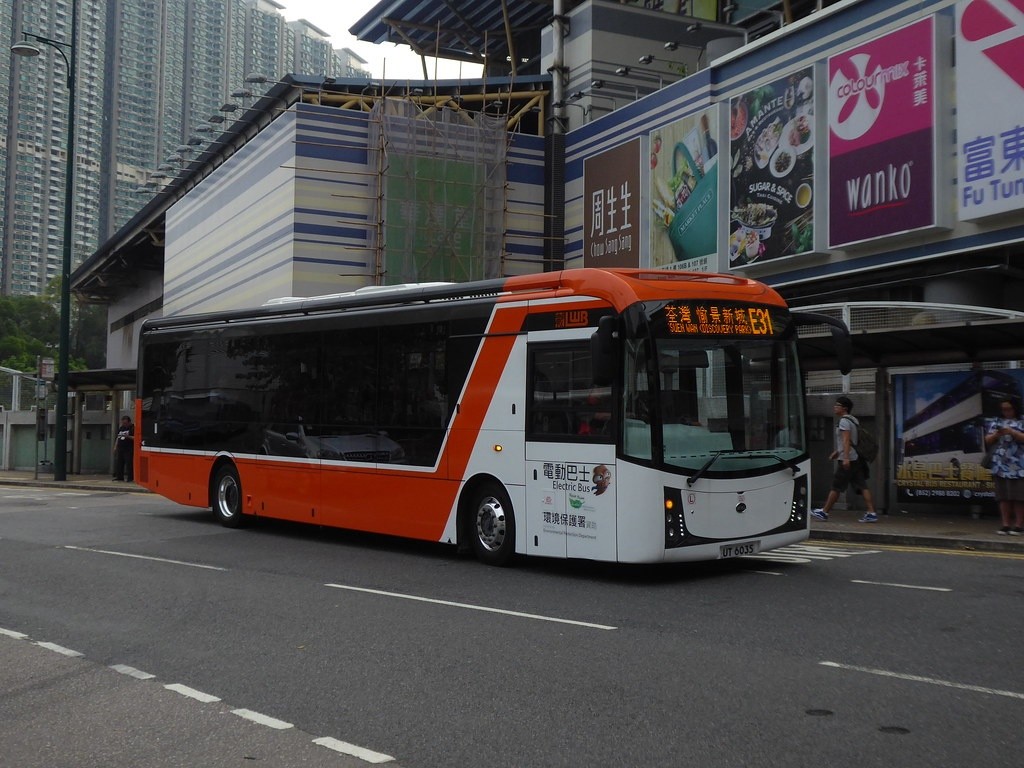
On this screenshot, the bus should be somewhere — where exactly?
[133,263,855,568]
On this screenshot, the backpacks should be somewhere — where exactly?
[838,417,878,463]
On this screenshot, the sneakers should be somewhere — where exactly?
[857,512,878,523]
[1008,527,1024,536]
[810,508,829,522]
[997,526,1009,535]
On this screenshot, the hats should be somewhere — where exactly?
[836,397,854,414]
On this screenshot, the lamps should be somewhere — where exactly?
[324,75,336,83]
[413,88,423,96]
[195,124,212,132]
[454,97,463,103]
[615,68,628,77]
[639,56,652,64]
[370,83,380,90]
[686,23,702,33]
[531,106,541,113]
[552,100,563,109]
[591,81,604,89]
[570,93,582,101]
[244,73,267,83]
[723,4,739,13]
[136,135,206,194]
[664,42,678,51]
[219,103,237,112]
[208,115,224,123]
[493,101,502,108]
[230,88,252,98]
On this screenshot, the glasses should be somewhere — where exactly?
[832,403,845,407]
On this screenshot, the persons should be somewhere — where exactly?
[112,416,134,482]
[591,393,635,434]
[983,398,1024,536]
[809,396,878,523]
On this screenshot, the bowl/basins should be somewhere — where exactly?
[737,210,778,241]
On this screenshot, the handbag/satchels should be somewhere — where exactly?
[981,443,995,469]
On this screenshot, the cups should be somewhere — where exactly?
[796,182,812,208]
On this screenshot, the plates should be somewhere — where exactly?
[779,114,814,155]
[770,145,797,177]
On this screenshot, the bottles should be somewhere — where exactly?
[698,114,718,176]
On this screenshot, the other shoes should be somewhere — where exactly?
[126,479,133,482]
[112,478,124,482]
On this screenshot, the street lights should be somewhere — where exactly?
[11,30,79,480]
